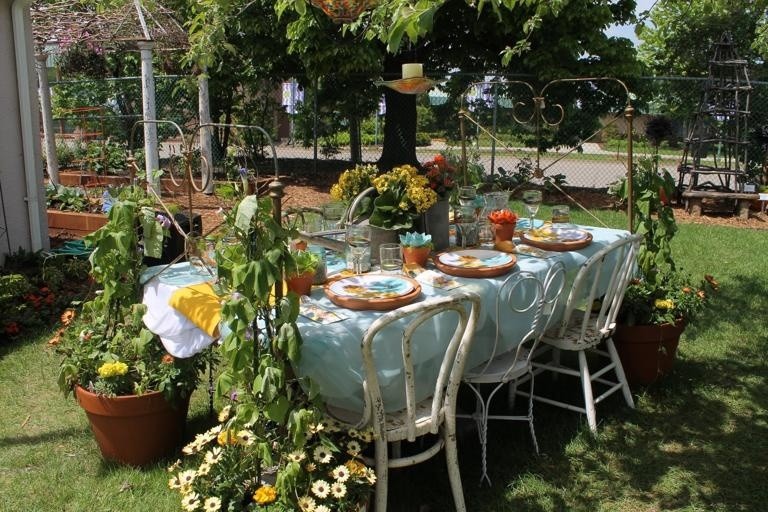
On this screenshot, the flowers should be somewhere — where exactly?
[619,263,721,327]
[173,394,377,512]
[333,151,457,225]
[37,301,209,391]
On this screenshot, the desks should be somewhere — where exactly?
[139,220,636,465]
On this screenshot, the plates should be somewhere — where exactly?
[438,250,515,269]
[331,276,415,299]
[523,225,588,243]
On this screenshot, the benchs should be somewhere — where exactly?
[681,190,760,221]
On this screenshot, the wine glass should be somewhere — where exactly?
[522,190,542,228]
[453,208,476,250]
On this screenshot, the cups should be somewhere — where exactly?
[458,185,476,206]
[552,205,570,228]
[344,225,373,275]
[379,244,404,275]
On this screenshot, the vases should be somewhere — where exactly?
[616,324,686,386]
[73,377,182,467]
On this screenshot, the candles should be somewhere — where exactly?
[401,62,424,78]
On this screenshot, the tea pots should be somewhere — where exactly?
[478,191,517,245]
